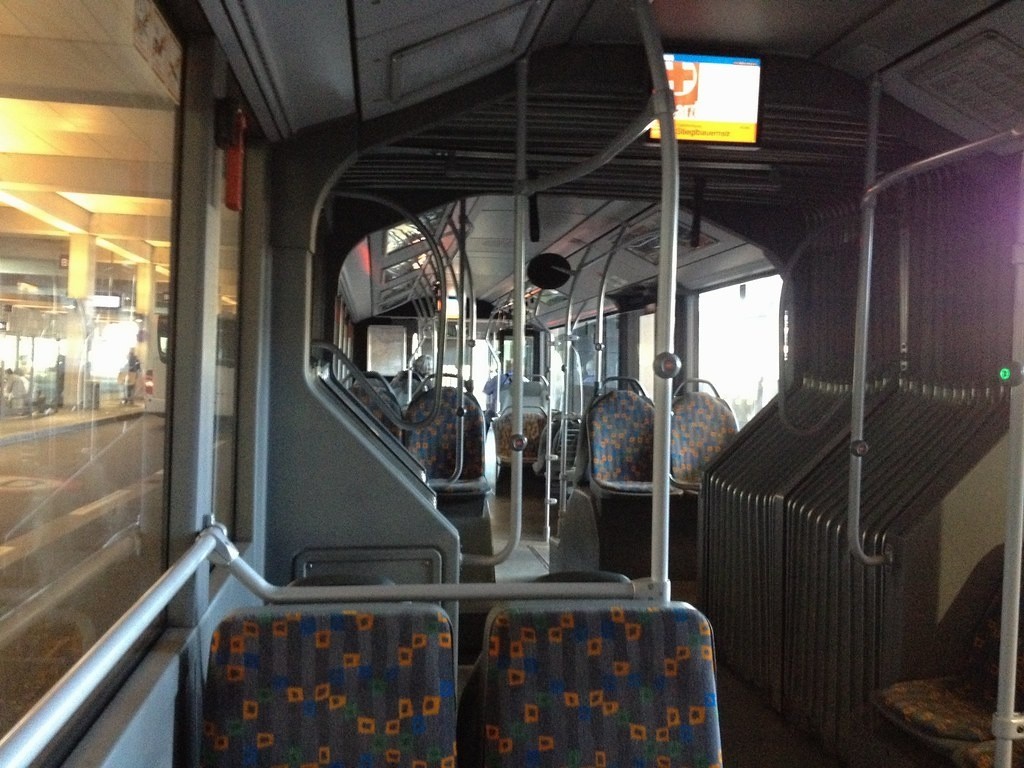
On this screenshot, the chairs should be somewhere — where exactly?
[587,379,683,498]
[873,545,1024,768]
[200,604,456,768]
[405,375,491,495]
[483,600,722,768]
[492,406,582,484]
[670,379,739,498]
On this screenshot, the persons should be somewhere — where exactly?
[399,353,434,393]
[482,359,514,430]
[5,347,139,411]
[582,358,596,385]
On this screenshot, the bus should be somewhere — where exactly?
[147,310,243,421]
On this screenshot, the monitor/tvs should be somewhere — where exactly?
[645,48,766,151]
